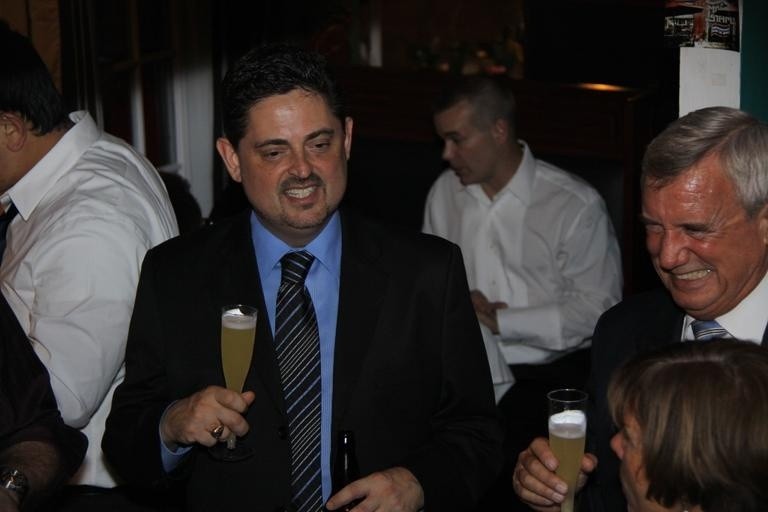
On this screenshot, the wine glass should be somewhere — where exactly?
[209,301,259,463]
[547,389,588,511]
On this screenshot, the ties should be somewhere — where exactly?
[690,319,727,342]
[0,202,19,267]
[273,249,323,511]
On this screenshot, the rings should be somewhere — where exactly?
[210,424,224,437]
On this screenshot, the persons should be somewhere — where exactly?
[96,40,497,510]
[511,106,767,510]
[603,336,766,511]
[0,294,89,511]
[1,22,178,492]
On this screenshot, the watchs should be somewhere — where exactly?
[2,468,29,497]
